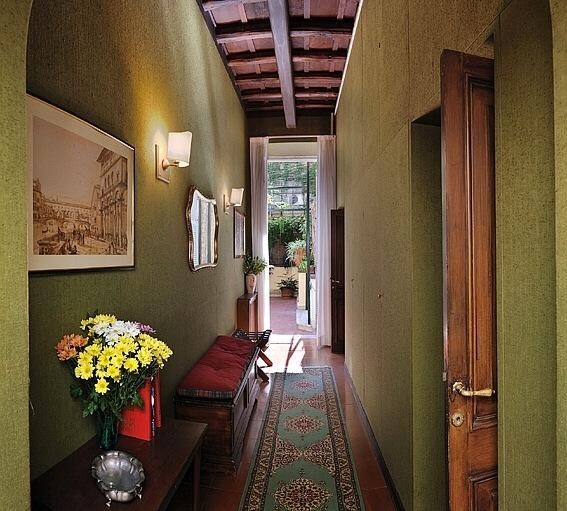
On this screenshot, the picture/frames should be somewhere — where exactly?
[25,91,137,277]
[232,207,245,258]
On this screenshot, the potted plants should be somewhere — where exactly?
[282,238,315,275]
[241,254,267,294]
[275,275,298,299]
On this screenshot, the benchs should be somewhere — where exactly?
[170,334,260,480]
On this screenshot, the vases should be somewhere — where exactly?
[92,410,119,450]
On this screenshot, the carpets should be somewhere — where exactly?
[238,365,365,511]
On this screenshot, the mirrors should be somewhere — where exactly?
[185,185,220,272]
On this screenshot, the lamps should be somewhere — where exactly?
[153,131,193,182]
[223,188,243,215]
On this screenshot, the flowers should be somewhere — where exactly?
[52,308,174,446]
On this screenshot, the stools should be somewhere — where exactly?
[231,329,272,383]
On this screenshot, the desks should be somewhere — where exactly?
[29,417,208,511]
